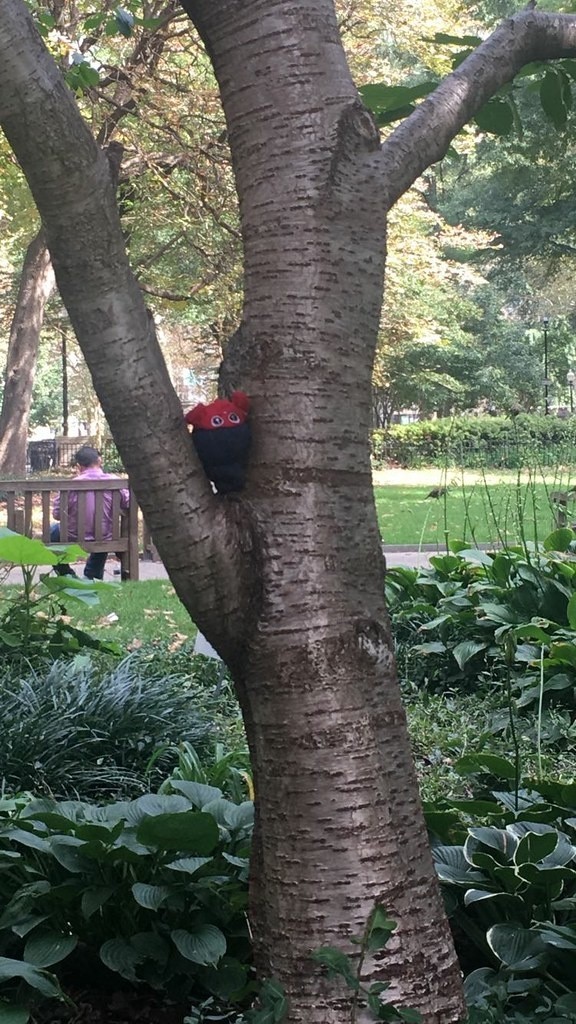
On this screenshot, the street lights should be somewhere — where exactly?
[566,368,575,412]
[541,316,550,414]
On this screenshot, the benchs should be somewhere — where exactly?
[0,478,139,581]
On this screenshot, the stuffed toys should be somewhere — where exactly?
[186,390,254,497]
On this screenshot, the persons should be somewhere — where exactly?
[43,447,131,581]
